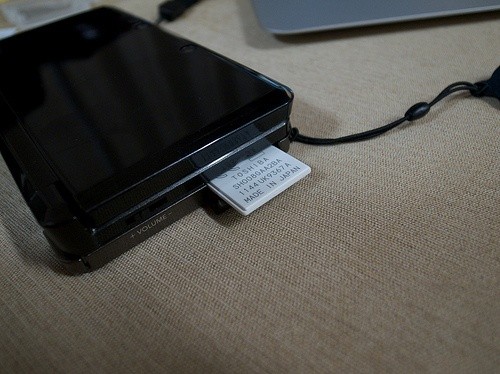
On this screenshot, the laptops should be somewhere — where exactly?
[249,0,487,37]
